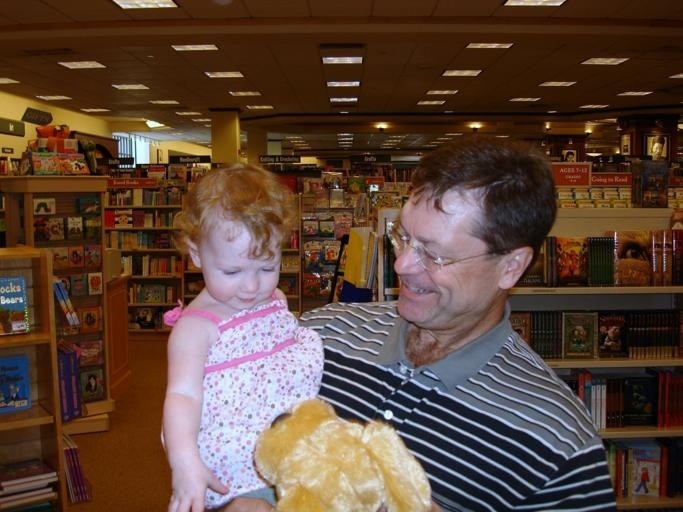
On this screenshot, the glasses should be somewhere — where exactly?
[386,219,512,273]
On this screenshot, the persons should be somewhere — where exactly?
[649,135,664,161]
[221,133,620,512]
[159,155,324,512]
[564,151,574,162]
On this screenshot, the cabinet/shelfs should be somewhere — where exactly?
[0,129,683,512]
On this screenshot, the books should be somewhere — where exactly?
[555,188,631,208]
[1,182,202,512]
[511,311,683,499]
[519,229,683,287]
[277,170,398,303]
